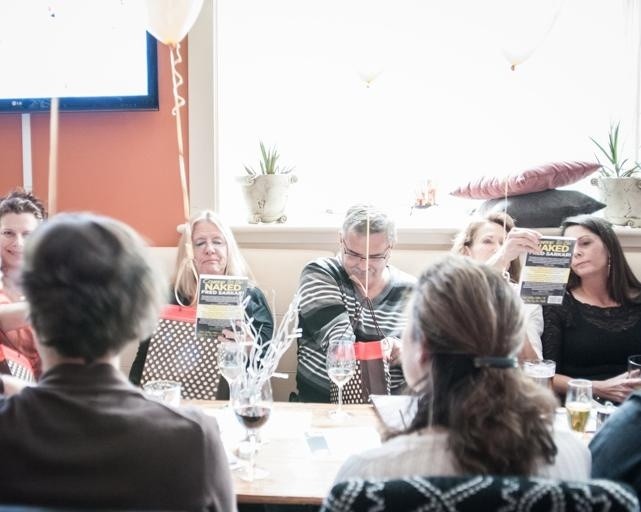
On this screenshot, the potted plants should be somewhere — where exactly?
[234,141,297,224]
[588,121,641,226]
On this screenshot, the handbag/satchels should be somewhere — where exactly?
[329,339,394,405]
[0,345,37,393]
[139,304,223,401]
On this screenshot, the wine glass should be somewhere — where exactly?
[218,341,246,412]
[628,355,641,389]
[326,340,356,421]
[565,380,592,433]
[231,376,273,483]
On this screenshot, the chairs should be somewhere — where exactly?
[319,477,641,512]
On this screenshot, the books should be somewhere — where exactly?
[516,235,577,307]
[192,270,247,343]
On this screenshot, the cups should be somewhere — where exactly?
[143,381,181,408]
[524,360,556,392]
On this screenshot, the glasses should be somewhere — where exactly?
[343,234,391,262]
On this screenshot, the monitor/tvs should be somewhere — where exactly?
[0,2,161,113]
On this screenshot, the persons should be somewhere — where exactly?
[515,213,641,403]
[590,390,640,493]
[0,211,237,512]
[0,191,49,401]
[397,212,555,395]
[130,209,274,401]
[321,257,592,482]
[292,206,420,403]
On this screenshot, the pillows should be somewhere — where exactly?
[468,188,607,228]
[447,162,600,199]
